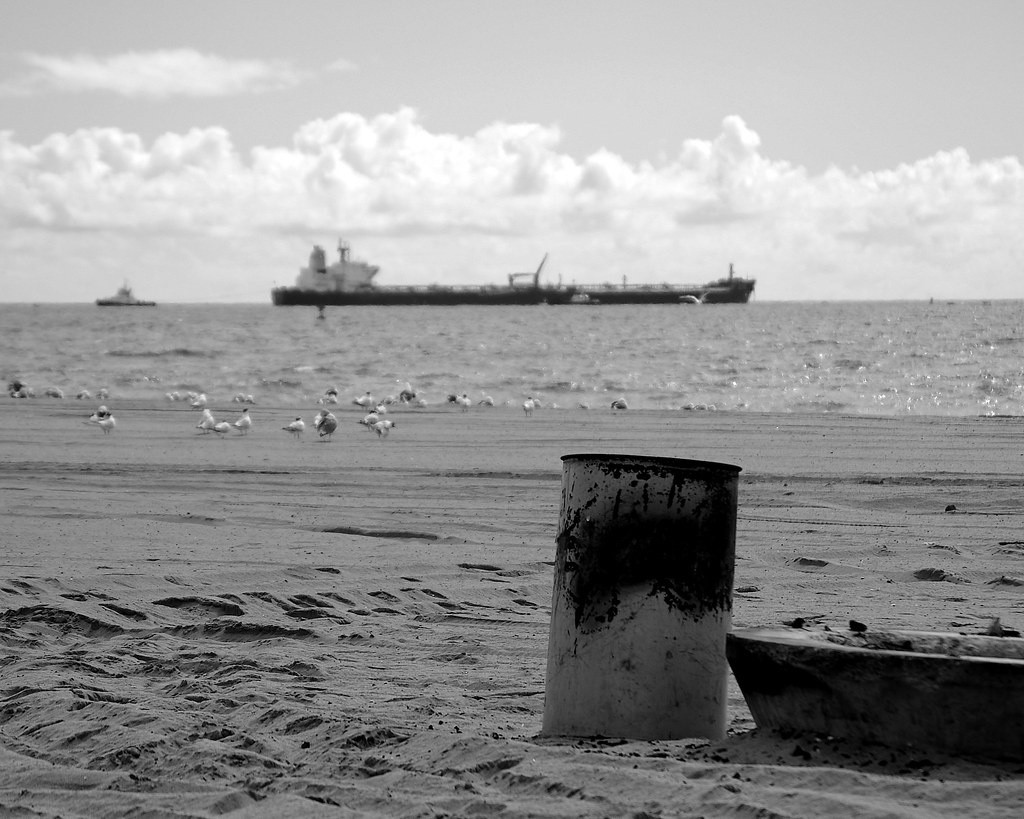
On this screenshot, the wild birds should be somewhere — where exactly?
[448,394,471,411]
[478,396,495,405]
[611,397,629,409]
[551,402,559,408]
[679,403,716,410]
[282,416,304,438]
[505,399,512,407]
[81,406,115,435]
[679,292,710,305]
[522,397,541,416]
[72,390,91,399]
[165,391,256,436]
[576,401,591,409]
[96,389,109,399]
[352,382,427,438]
[312,408,338,442]
[317,388,337,406]
[7,381,63,399]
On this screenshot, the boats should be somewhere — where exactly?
[94,277,156,306]
[270,236,754,304]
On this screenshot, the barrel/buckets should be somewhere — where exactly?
[541,453,743,742]
